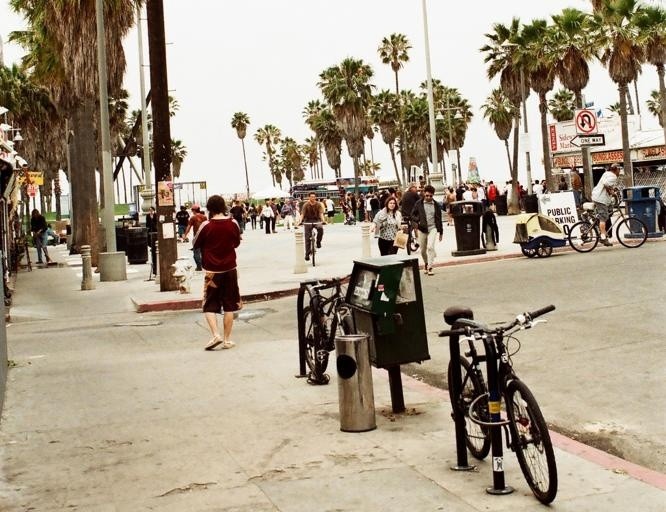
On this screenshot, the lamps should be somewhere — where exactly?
[6,126,24,142]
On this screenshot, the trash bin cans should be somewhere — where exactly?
[622,185,665,237]
[334,335,376,432]
[125,227,148,264]
[449,201,486,256]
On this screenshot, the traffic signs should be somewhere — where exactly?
[569,134,607,148]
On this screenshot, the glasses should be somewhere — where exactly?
[426,195,432,197]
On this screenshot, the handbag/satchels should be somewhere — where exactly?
[393,229,409,249]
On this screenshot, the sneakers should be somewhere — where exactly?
[599,238,613,246]
[305,255,309,260]
[428,268,434,275]
[317,240,321,248]
[424,263,429,274]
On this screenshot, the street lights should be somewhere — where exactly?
[433,94,463,198]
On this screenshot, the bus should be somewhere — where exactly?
[335,176,379,191]
[290,180,338,198]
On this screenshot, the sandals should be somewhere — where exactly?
[204,335,223,350]
[223,342,236,349]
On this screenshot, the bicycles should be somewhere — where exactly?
[301,220,325,268]
[566,193,650,255]
[297,271,356,381]
[402,217,421,257]
[437,302,559,506]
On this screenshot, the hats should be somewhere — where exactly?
[611,163,624,169]
[190,205,200,210]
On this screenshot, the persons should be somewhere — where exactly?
[445,180,496,226]
[592,161,622,249]
[557,177,569,192]
[300,190,324,261]
[30,209,53,263]
[47,222,58,246]
[190,194,242,351]
[325,196,335,224]
[181,203,208,272]
[174,206,205,242]
[570,166,583,209]
[145,206,158,252]
[380,182,425,237]
[408,185,444,276]
[369,197,407,255]
[229,196,301,234]
[338,187,377,224]
[503,180,547,209]
